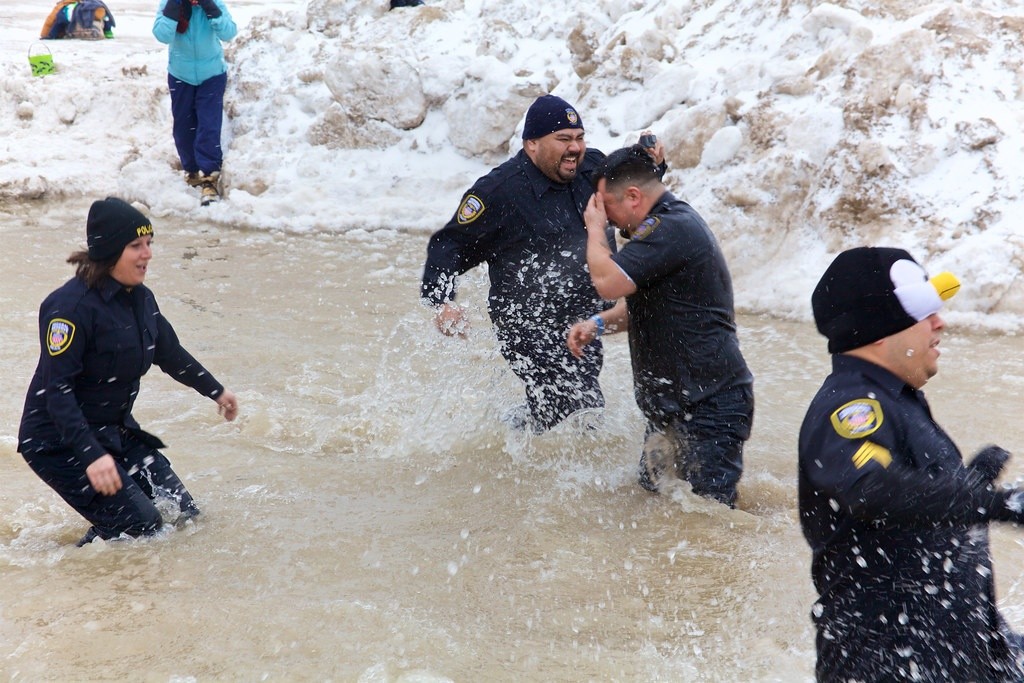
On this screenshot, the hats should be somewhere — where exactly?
[524,95,586,142]
[86,196,154,260]
[810,247,963,355]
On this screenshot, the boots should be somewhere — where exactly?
[199,172,223,205]
[184,169,201,188]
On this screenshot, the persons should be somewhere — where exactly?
[16,194,239,548]
[564,147,755,510]
[150,0,238,204]
[418,94,667,443]
[797,247,1024,682]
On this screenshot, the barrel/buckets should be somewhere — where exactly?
[26,39,56,77]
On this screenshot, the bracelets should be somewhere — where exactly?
[591,315,605,338]
